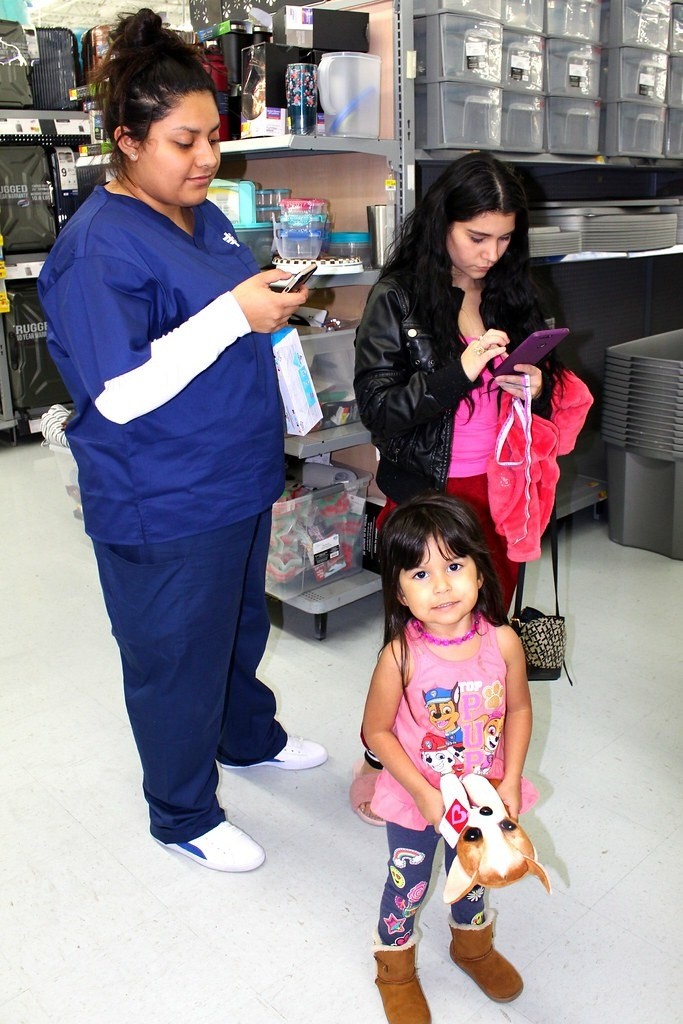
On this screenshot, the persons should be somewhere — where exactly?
[348,152,562,827]
[36,8,330,874]
[369,491,540,1024]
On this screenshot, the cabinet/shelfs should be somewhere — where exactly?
[0,109,119,439]
[178,1,682,640]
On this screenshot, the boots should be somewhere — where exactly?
[371,926,432,1024]
[447,907,524,1003]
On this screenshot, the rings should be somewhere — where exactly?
[473,347,486,355]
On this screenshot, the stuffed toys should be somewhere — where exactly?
[439,772,552,905]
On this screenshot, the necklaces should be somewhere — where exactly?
[413,609,483,646]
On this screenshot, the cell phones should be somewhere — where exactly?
[284,264,318,294]
[493,327,570,378]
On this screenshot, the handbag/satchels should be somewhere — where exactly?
[509,606,574,686]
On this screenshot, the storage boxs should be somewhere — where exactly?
[186,3,683,600]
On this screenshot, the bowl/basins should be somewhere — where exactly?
[255,189,371,260]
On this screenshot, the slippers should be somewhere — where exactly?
[348,758,387,828]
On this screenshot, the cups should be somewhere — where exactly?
[286,63,318,136]
[317,52,382,139]
[367,205,395,268]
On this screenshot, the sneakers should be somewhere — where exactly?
[214,733,329,771]
[147,817,266,873]
